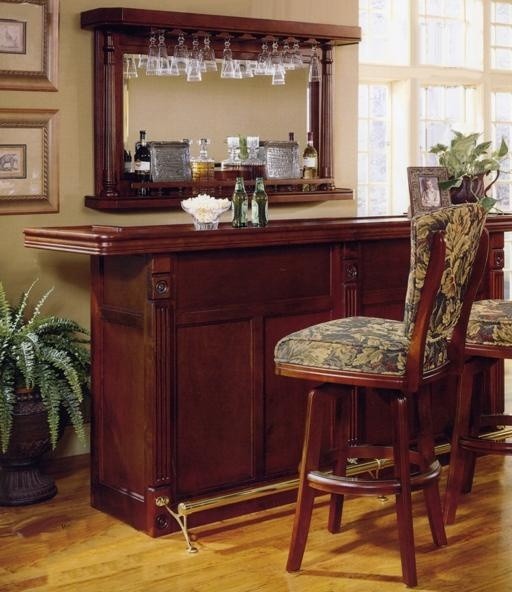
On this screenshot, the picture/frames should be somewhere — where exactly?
[0,0,61,216]
[406,166,451,217]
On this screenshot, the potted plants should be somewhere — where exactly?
[429,129,508,213]
[0,277,93,507]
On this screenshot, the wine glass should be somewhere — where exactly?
[123,26,323,86]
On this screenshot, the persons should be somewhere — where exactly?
[421,177,440,206]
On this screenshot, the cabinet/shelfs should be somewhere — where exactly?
[22,214,512,539]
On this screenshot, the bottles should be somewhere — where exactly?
[232,176,248,228]
[124,129,152,196]
[251,177,269,227]
[221,136,265,196]
[287,131,318,192]
[193,138,215,197]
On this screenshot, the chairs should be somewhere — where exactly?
[274,203,494,586]
[441,297,510,526]
